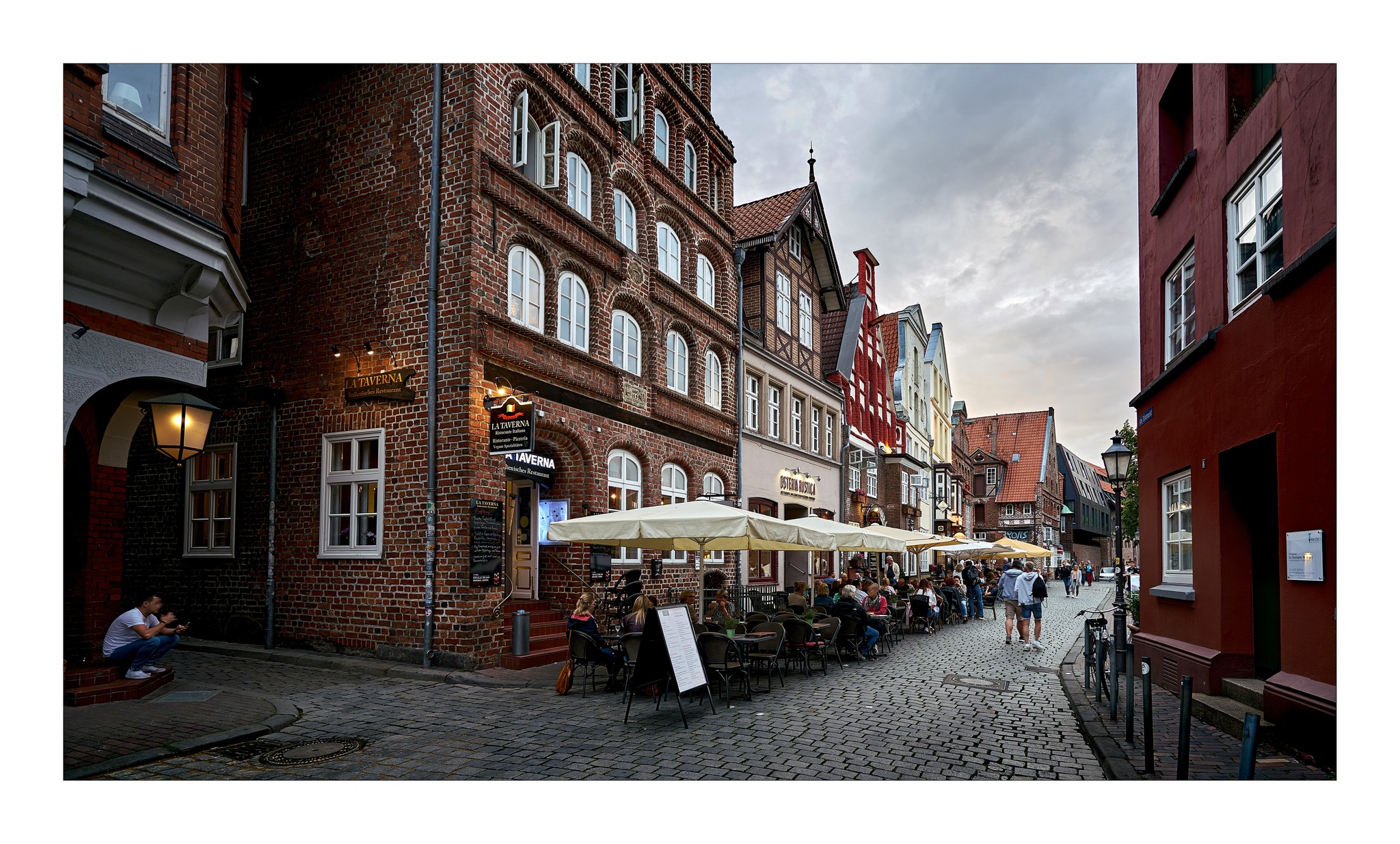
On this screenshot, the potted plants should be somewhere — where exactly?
[803,604,821,624]
[775,604,789,612]
[907,587,915,599]
[724,613,738,637]
[888,594,899,608]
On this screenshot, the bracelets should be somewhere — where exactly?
[161,620,168,625]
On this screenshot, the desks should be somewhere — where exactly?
[776,622,831,671]
[901,598,941,630]
[599,634,626,687]
[888,606,906,643]
[828,614,893,660]
[721,631,776,699]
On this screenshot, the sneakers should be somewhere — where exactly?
[142,665,166,673]
[968,616,974,620]
[125,669,151,679]
[979,616,986,620]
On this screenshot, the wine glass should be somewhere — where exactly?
[615,623,620,637]
[805,594,810,606]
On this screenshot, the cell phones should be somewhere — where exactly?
[180,622,191,631]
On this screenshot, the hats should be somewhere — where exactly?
[856,570,864,573]
[1072,559,1074,561]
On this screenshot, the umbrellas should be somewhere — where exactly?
[545,495,838,625]
[906,530,980,587]
[862,522,943,588]
[785,514,907,607]
[924,537,1054,569]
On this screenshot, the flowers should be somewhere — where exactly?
[929,564,937,568]
[854,488,867,496]
[733,608,741,619]
[851,553,865,560]
[902,503,921,511]
[361,530,376,540]
[339,527,350,536]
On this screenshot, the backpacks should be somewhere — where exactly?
[1032,573,1048,609]
[966,566,981,586]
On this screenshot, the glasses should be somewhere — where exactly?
[898,583,904,585]
[843,576,848,578]
[687,596,696,599]
[590,603,596,607]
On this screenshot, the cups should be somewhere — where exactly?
[940,574,941,577]
[706,617,711,622]
[711,601,716,609]
[839,596,843,598]
[621,618,624,627]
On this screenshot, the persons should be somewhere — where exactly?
[814,583,834,615]
[821,556,1012,634]
[861,583,890,654]
[1058,561,1073,597]
[1085,560,1095,586]
[997,560,1027,643]
[623,595,651,660]
[1071,559,1077,568]
[103,593,188,679]
[680,590,699,624]
[1079,561,1087,586]
[568,594,626,692]
[647,596,657,607]
[706,589,735,623]
[1066,560,1071,566]
[1070,565,1082,599]
[1014,561,1049,651]
[788,581,808,609]
[831,585,878,660]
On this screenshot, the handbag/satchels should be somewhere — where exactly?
[1068,584,1073,592]
[554,660,574,694]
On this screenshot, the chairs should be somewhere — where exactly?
[565,569,1000,709]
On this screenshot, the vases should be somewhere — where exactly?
[902,507,922,517]
[734,618,740,624]
[852,493,868,504]
[929,567,937,571]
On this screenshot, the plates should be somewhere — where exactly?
[867,613,874,614]
[870,615,888,617]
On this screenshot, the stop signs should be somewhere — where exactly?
[1057,547,1063,554]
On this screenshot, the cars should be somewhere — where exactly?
[1098,567,1116,582]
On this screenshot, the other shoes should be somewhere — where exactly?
[929,626,935,632]
[854,651,866,660]
[605,679,625,693]
[1032,641,1044,650]
[1066,594,1070,597]
[873,644,878,654]
[1071,595,1078,598]
[1024,645,1031,651]
[944,613,968,624]
[850,651,855,657]
[924,627,932,634]
[1005,636,1012,644]
[1019,636,1025,642]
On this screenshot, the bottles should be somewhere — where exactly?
[932,583,938,588]
[986,582,988,586]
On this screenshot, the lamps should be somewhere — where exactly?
[935,497,964,518]
[785,468,821,483]
[135,384,223,467]
[880,445,884,450]
[890,447,898,453]
[495,376,529,402]
[63,311,91,340]
[330,344,360,377]
[364,340,398,370]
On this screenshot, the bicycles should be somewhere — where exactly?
[1074,603,1132,702]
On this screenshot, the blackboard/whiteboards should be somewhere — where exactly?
[470,499,502,588]
[589,510,614,582]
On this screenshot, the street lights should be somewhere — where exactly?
[1134,528,1139,568]
[1100,429,1134,675]
[1046,537,1051,582]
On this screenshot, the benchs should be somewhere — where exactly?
[1042,573,1052,588]
